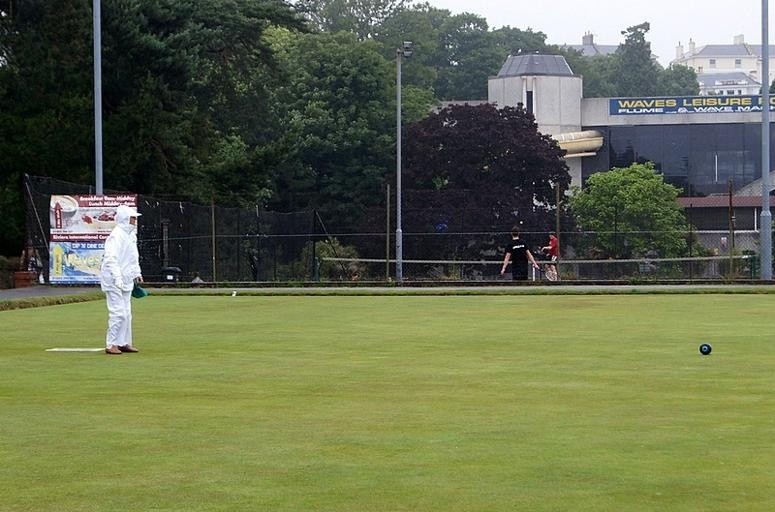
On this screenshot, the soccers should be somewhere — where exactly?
[700,344,711,354]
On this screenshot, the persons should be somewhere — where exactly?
[541,232,560,281]
[101,204,147,354]
[500,225,541,284]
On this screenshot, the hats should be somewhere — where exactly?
[117,205,142,216]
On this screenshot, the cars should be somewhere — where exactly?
[138,252,183,289]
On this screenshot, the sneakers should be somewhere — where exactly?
[106,346,121,353]
[118,344,137,351]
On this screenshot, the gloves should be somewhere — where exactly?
[115,279,123,288]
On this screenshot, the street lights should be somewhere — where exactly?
[394,39,414,283]
